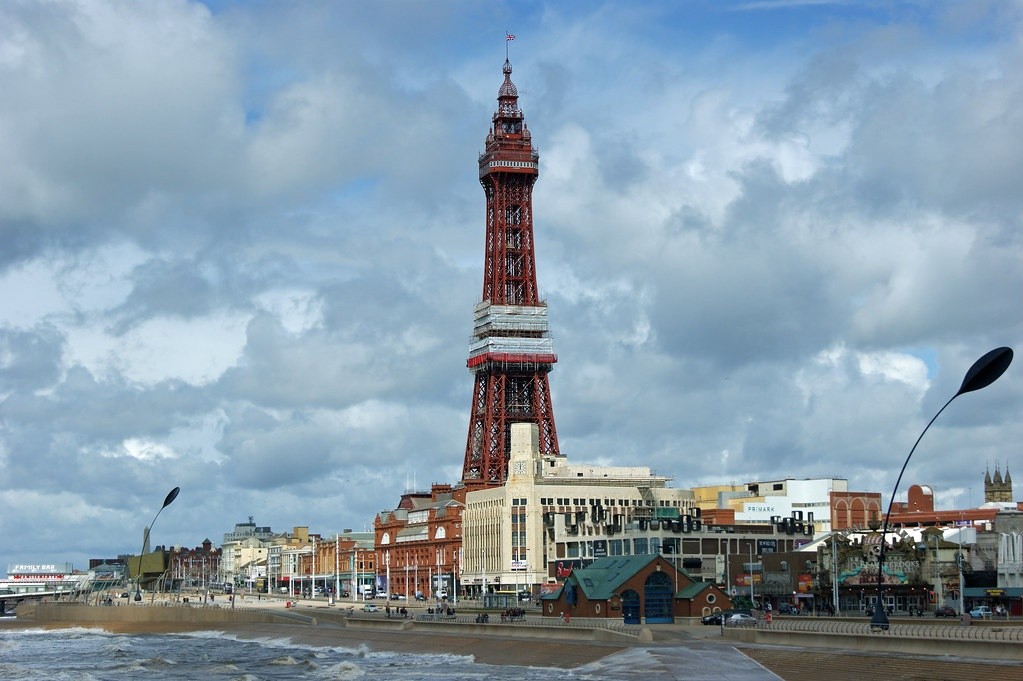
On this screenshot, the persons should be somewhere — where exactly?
[426,607,451,615]
[506,607,525,619]
[919,608,925,617]
[828,605,835,617]
[909,605,913,616]
[400,607,408,617]
[484,613,489,623]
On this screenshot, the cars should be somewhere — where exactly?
[364,604,380,612]
[279,585,460,603]
[934,606,956,617]
[701,609,752,625]
[726,613,757,627]
[968,605,993,619]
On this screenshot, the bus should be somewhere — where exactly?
[207,582,233,595]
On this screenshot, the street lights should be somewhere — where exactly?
[669,546,674,565]
[590,547,594,563]
[722,541,730,597]
[829,533,840,617]
[374,552,378,598]
[869,345,1016,631]
[482,549,486,596]
[133,487,181,602]
[746,542,753,605]
[527,548,532,603]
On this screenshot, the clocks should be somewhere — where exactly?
[515,462,527,474]
